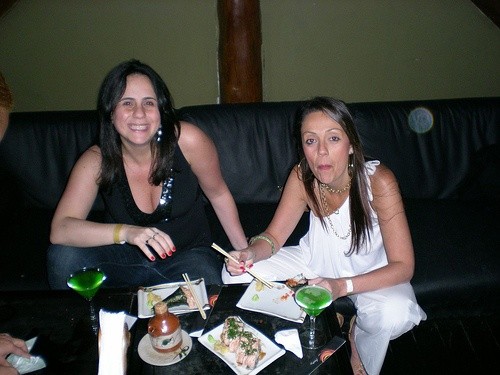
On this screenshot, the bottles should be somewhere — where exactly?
[147,302,182,353]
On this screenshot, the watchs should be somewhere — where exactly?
[342,277,354,296]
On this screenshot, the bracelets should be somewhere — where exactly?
[248,236,275,259]
[112,223,126,245]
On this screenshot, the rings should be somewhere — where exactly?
[224,257,229,265]
[146,237,153,244]
[151,233,159,238]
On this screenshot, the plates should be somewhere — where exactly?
[137,278,210,319]
[236,278,307,323]
[198,316,286,375]
[137,329,193,366]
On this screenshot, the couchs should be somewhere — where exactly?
[0,94,500,318]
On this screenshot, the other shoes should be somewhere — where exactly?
[348,315,370,375]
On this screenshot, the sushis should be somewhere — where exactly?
[151,286,195,308]
[221,316,262,369]
[286,274,308,292]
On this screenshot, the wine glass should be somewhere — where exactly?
[65,266,108,335]
[294,284,334,350]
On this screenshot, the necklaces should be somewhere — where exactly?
[316,170,354,239]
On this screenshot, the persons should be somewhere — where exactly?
[0,333,32,375]
[221,94,427,375]
[48,58,249,292]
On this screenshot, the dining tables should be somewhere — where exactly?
[0,283,341,375]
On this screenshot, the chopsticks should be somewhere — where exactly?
[182,272,207,320]
[211,243,273,289]
